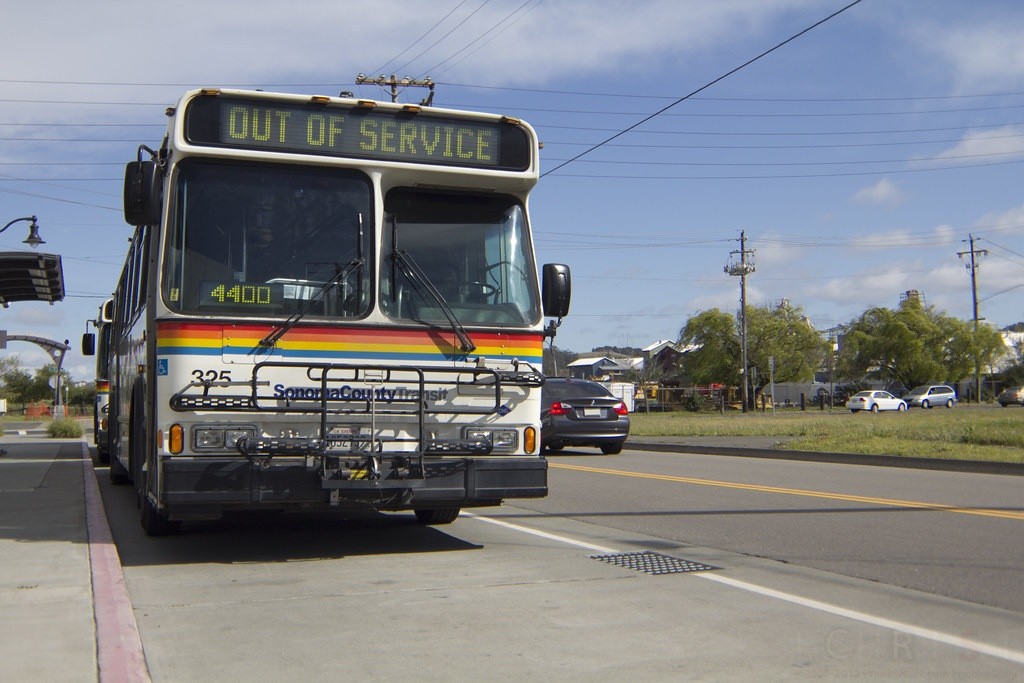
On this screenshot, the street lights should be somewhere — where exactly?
[65,386,68,415]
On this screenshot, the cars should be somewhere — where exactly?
[540,377,630,455]
[998,386,1024,407]
[846,390,907,413]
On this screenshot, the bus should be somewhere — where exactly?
[82,88,571,536]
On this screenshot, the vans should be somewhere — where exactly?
[902,385,957,409]
